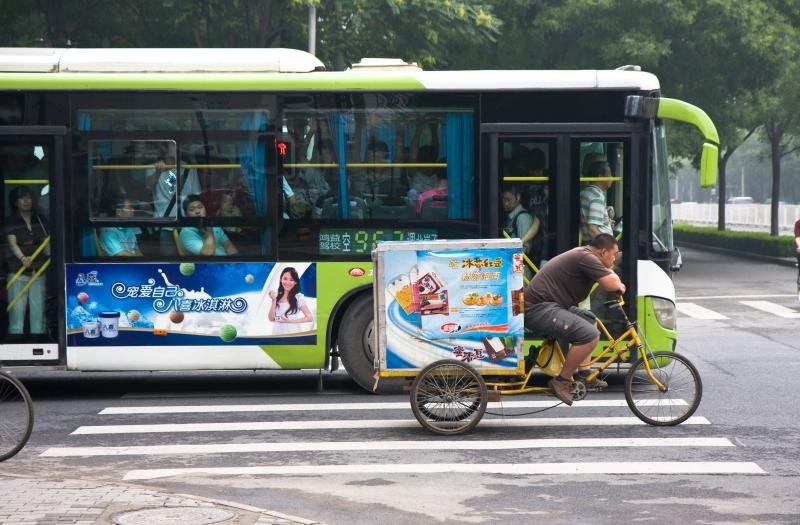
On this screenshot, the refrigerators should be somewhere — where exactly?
[372,238,523,372]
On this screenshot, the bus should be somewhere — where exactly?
[0,50,720,394]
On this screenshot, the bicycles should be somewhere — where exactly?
[0,368,35,463]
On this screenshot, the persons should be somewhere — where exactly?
[794,220,800,292]
[521,233,625,407]
[268,266,313,323]
[577,151,616,249]
[6,187,52,337]
[96,95,457,259]
[501,148,550,285]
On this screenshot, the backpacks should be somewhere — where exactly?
[513,210,549,268]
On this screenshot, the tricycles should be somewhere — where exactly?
[406,294,703,436]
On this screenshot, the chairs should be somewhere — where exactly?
[94,224,185,259]
[325,195,449,220]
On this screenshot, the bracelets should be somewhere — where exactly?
[18,255,26,261]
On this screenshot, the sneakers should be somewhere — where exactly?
[579,375,609,388]
[549,378,574,406]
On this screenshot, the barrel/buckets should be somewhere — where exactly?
[99,312,120,337]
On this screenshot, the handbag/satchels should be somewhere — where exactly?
[145,230,161,256]
[536,339,565,376]
[41,237,50,256]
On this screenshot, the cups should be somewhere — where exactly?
[82,322,100,338]
